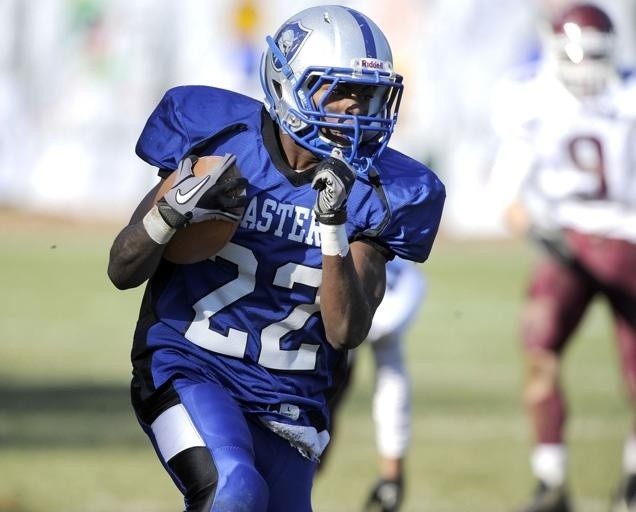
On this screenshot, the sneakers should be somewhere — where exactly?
[525,481,571,510]
[360,480,404,512]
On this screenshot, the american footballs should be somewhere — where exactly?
[153,155,246,264]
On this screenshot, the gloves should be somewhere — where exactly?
[155,153,251,231]
[310,148,356,225]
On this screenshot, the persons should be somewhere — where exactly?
[104,3,448,510]
[315,249,429,511]
[479,6,636,510]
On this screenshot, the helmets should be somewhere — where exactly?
[259,3,404,172]
[549,5,614,101]
